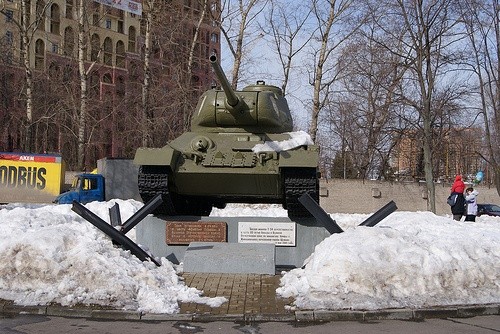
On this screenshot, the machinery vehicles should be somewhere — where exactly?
[133,52,322,221]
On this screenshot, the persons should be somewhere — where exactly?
[464,188,478,222]
[450,175,465,193]
[446,192,466,221]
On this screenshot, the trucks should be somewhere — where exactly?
[0,153,65,205]
[55,156,144,205]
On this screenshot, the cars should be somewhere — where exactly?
[476,204,500,217]
